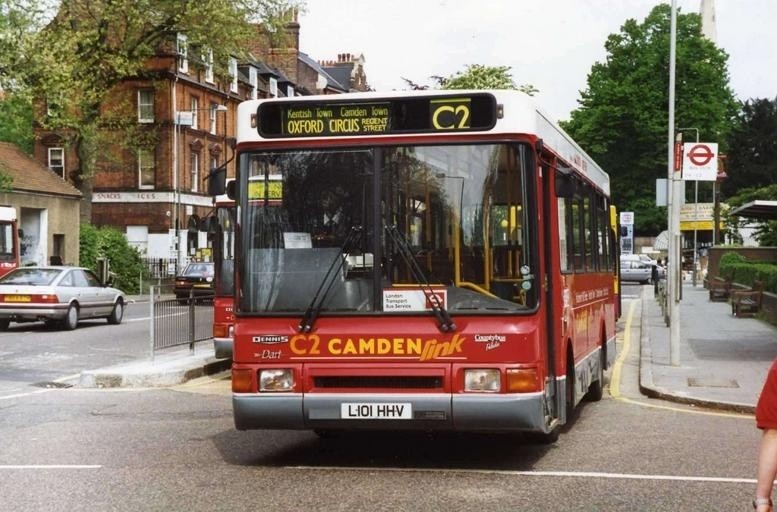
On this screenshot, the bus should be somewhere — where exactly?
[202,84,623,444]
[200,173,283,360]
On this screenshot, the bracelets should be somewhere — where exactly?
[751,498,773,509]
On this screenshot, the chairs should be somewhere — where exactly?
[413,246,523,302]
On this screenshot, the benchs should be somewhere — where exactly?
[709,274,763,319]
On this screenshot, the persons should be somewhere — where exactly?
[652,257,663,294]
[751,356,776,512]
[663,256,668,266]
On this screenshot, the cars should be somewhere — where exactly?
[172,262,215,304]
[616,256,668,284]
[0,264,128,331]
